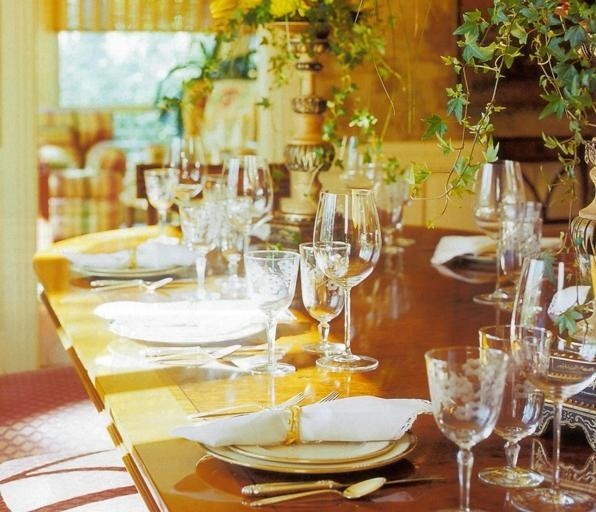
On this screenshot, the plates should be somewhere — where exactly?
[197,430,417,475]
[59,237,191,280]
[102,296,272,346]
[460,237,563,263]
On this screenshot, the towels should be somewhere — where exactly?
[168,393,435,450]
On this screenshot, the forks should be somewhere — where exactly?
[187,388,343,423]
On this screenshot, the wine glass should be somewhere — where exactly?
[424,346,509,512]
[298,242,349,355]
[477,324,554,488]
[242,250,297,375]
[339,135,415,255]
[472,161,542,305]
[311,188,382,373]
[143,134,272,306]
[508,254,596,512]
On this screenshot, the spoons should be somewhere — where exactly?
[240,476,388,508]
[88,274,173,293]
[134,344,242,367]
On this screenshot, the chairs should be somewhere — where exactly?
[486,133,590,227]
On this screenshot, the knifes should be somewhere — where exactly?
[240,472,447,498]
